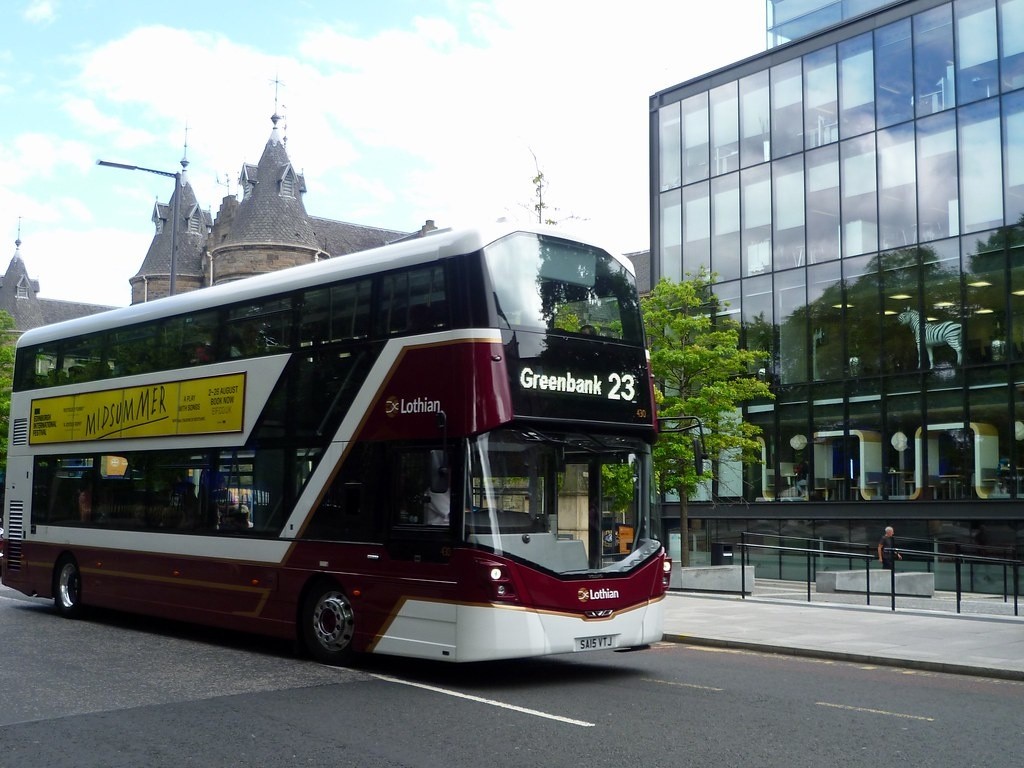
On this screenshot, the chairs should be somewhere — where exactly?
[34,358,141,389]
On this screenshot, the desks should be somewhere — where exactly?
[829,478,845,501]
[939,475,965,500]
[888,472,914,495]
[780,475,799,497]
[1001,468,1024,494]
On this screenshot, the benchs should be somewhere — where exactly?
[982,479,998,494]
[815,486,829,501]
[768,482,776,497]
[849,476,860,500]
[866,481,880,496]
[801,474,809,496]
[928,484,940,499]
[904,470,915,495]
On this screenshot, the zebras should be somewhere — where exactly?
[898,309,962,370]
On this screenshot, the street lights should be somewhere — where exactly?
[97,159,182,297]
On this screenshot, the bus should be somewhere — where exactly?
[1,218,710,663]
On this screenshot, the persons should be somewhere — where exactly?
[579,325,597,336]
[424,465,471,527]
[796,461,808,497]
[79,484,93,520]
[878,526,902,572]
